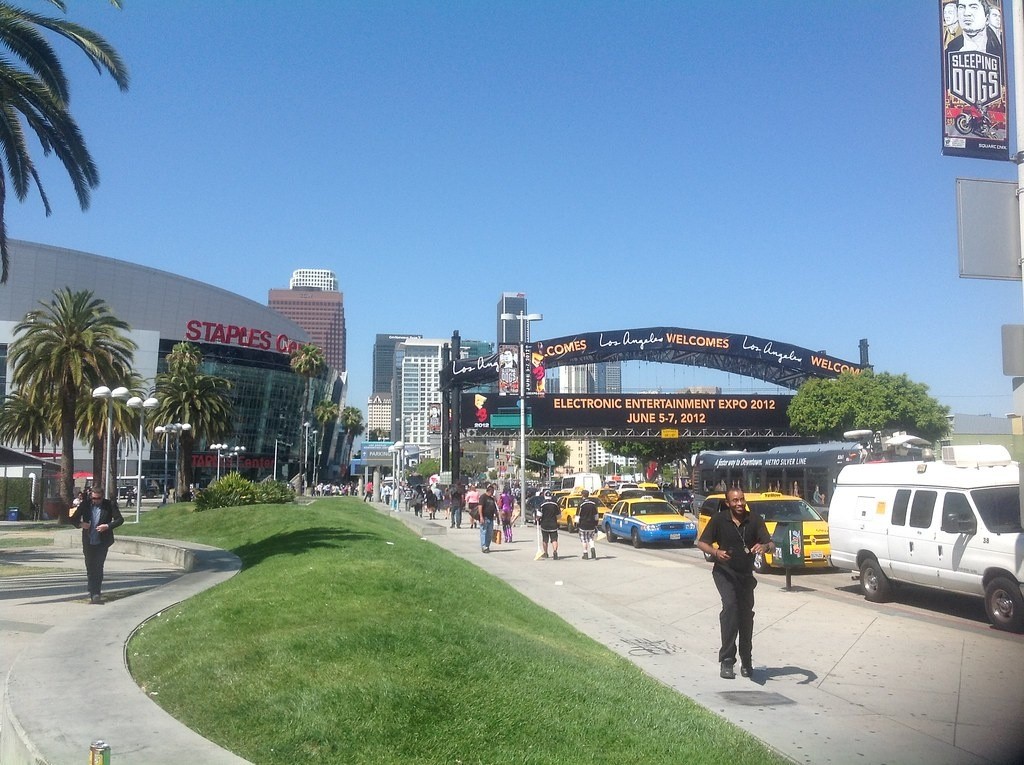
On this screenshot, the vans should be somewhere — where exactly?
[826,442,1024,637]
[696,491,838,573]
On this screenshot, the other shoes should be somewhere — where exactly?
[541,553,548,557]
[591,548,595,558]
[457,525,460,528]
[482,546,490,553]
[450,524,455,528]
[582,553,588,559]
[471,524,473,528]
[92,595,101,604]
[553,551,558,560]
[475,523,477,528]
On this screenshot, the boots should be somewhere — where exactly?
[429,514,432,520]
[432,513,435,518]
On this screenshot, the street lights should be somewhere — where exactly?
[395,441,405,512]
[388,445,398,508]
[154,422,192,505]
[304,421,312,495]
[313,430,318,487]
[501,309,547,526]
[125,397,160,524]
[210,443,228,483]
[230,446,246,473]
[93,386,131,500]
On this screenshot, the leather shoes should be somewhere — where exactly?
[741,659,752,678]
[720,662,734,677]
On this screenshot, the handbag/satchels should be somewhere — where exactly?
[492,529,501,544]
[469,506,480,520]
[411,499,419,507]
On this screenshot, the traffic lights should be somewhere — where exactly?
[496,451,499,459]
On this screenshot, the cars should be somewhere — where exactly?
[545,485,619,507]
[555,493,611,532]
[605,479,694,514]
[601,495,699,547]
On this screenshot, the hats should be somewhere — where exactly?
[581,490,589,496]
[544,490,552,499]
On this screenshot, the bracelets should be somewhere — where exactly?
[765,543,770,553]
[715,549,717,557]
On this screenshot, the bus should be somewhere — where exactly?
[561,472,602,494]
[84,475,160,498]
[691,431,932,523]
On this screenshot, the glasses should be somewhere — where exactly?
[92,497,102,501]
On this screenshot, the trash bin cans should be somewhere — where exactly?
[8,507,19,521]
[771,521,805,564]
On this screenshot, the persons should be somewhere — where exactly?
[697,487,776,679]
[478,486,500,553]
[443,480,532,517]
[575,490,598,560]
[660,482,692,493]
[536,490,560,559]
[363,480,373,502]
[126,489,134,507]
[427,490,436,520]
[465,485,481,528]
[450,481,464,528]
[73,487,93,516]
[310,481,443,512]
[412,484,424,518]
[443,488,451,519]
[498,487,513,542]
[69,488,124,603]
